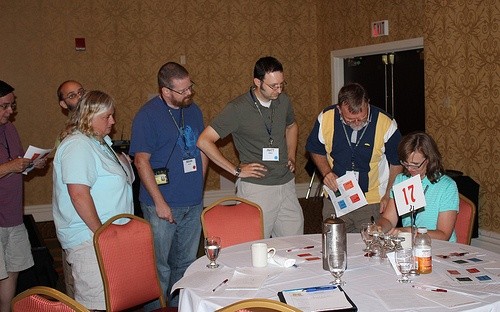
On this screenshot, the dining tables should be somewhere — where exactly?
[176,232,500,312]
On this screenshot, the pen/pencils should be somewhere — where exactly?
[371,216,375,225]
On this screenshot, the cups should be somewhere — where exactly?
[252,243,276,268]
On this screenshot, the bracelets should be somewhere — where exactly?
[235,167,241,178]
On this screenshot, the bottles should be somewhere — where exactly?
[381,234,395,264]
[368,231,384,264]
[412,227,432,274]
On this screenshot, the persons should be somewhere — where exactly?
[51,91,137,312]
[0,80,50,312]
[53,79,85,153]
[130,61,208,308]
[196,56,305,241]
[368,132,459,244]
[306,83,406,238]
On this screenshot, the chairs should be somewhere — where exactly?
[93,213,179,312]
[201,196,265,251]
[453,193,476,246]
[214,298,304,312]
[11,287,91,312]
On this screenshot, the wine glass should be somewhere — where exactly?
[396,249,414,284]
[204,236,221,269]
[328,250,348,286]
[361,223,377,252]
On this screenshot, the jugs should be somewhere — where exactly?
[323,213,348,272]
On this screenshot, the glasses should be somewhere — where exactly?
[341,103,369,125]
[164,80,196,95]
[0,104,16,110]
[260,79,288,90]
[62,89,87,100]
[400,159,427,170]
